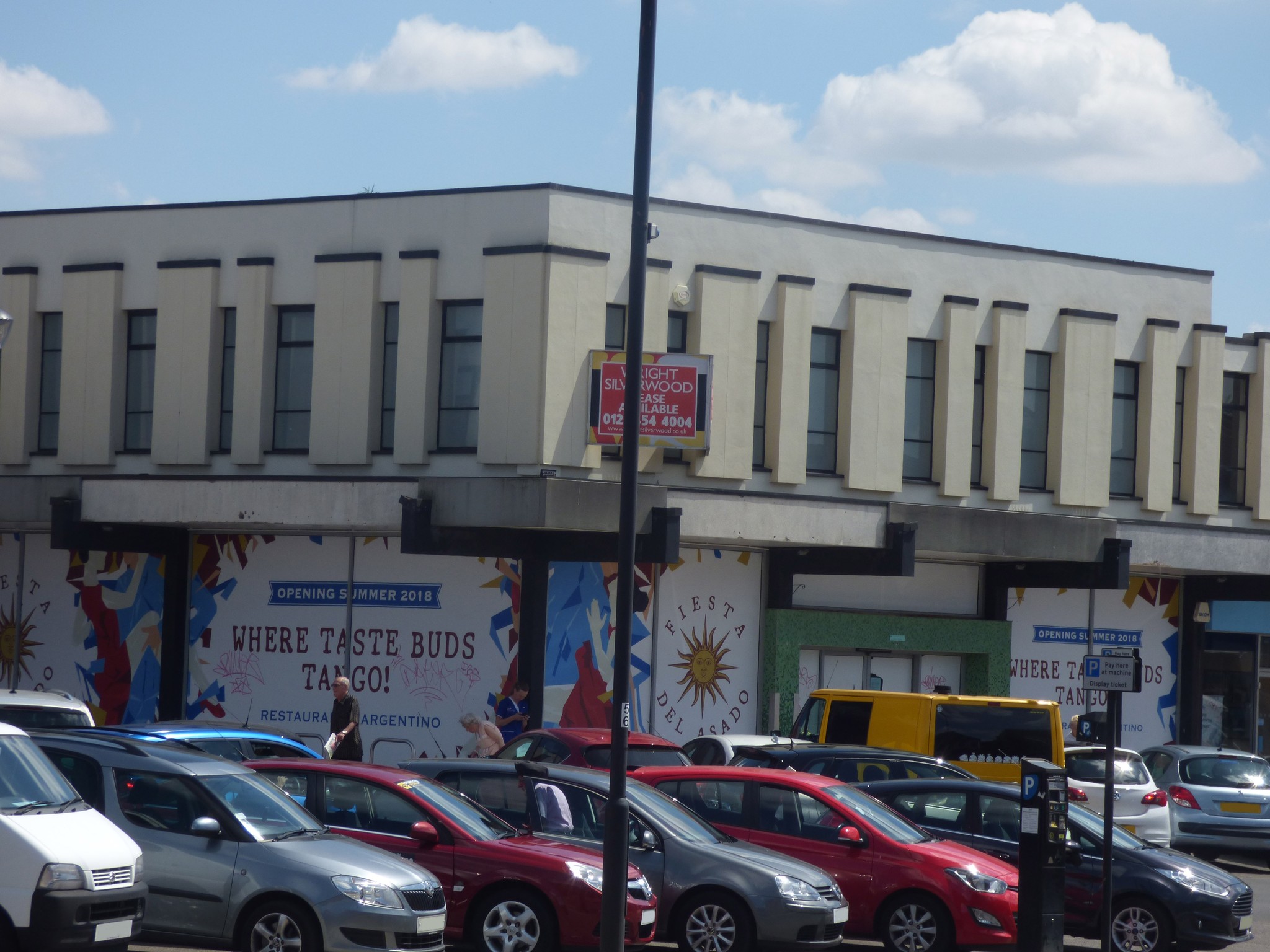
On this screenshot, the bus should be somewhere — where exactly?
[771,688,1064,784]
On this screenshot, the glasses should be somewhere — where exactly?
[330,684,345,688]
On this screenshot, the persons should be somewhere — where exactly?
[495,682,530,745]
[514,760,573,835]
[329,676,363,764]
[276,776,288,789]
[1064,715,1081,741]
[459,713,505,759]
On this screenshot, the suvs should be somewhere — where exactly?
[0,688,96,730]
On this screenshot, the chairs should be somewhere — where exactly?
[762,791,780,830]
[332,791,360,827]
[838,762,885,783]
[954,799,1012,841]
[570,807,594,839]
[130,776,170,828]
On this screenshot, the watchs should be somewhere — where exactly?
[342,730,346,735]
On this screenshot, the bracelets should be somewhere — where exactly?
[474,749,478,753]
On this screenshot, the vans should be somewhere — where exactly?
[0,724,147,952]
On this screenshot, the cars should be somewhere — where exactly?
[12,729,449,952]
[1064,742,1171,850]
[477,728,708,831]
[152,757,661,952]
[599,765,1018,952]
[814,776,1256,952]
[389,758,851,952]
[1132,744,1270,862]
[680,734,812,800]
[59,718,359,816]
[702,742,996,833]
